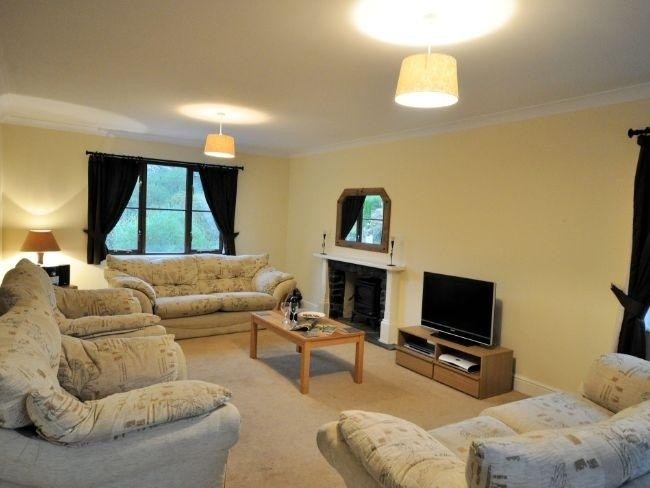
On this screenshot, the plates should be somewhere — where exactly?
[299,311,326,319]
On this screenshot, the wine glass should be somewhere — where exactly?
[280,300,300,325]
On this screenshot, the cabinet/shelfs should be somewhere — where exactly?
[394,322,515,402]
[56,284,78,289]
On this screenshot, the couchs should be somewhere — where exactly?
[0,294,242,488]
[312,349,650,488]
[0,257,168,342]
[101,253,298,344]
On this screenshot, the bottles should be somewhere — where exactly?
[290,288,298,323]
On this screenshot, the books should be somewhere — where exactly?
[290,318,340,336]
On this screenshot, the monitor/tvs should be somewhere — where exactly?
[420,270,496,347]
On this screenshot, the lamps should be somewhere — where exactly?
[204,111,237,159]
[17,229,62,266]
[394,31,459,113]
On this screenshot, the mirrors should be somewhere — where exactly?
[335,187,392,254]
